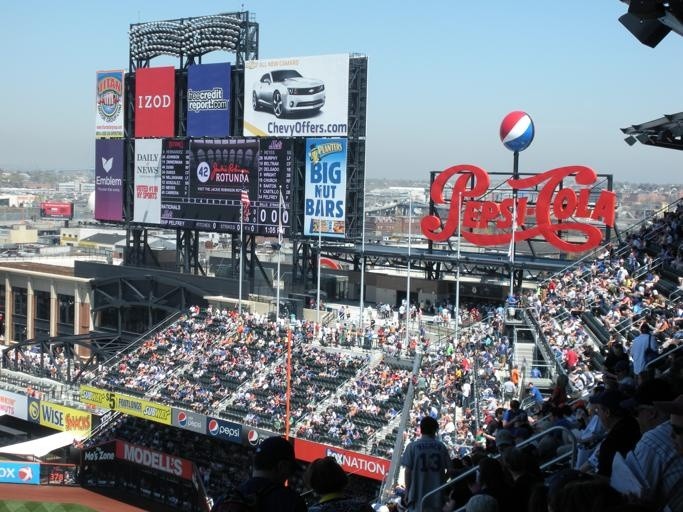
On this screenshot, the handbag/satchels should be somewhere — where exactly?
[644,348,660,363]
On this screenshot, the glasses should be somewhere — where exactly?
[668,422,683,435]
[634,408,652,417]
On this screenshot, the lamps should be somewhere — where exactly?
[624,132,648,146]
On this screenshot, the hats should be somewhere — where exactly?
[251,436,303,471]
[496,429,515,448]
[590,360,683,410]
[467,495,499,512]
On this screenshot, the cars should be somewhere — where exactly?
[252,69,325,118]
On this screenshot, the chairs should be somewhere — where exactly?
[76,210,683,463]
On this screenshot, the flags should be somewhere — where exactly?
[278,191,286,209]
[240,174,251,222]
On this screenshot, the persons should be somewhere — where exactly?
[504,200,682,300]
[0,334,112,386]
[91,298,682,512]
